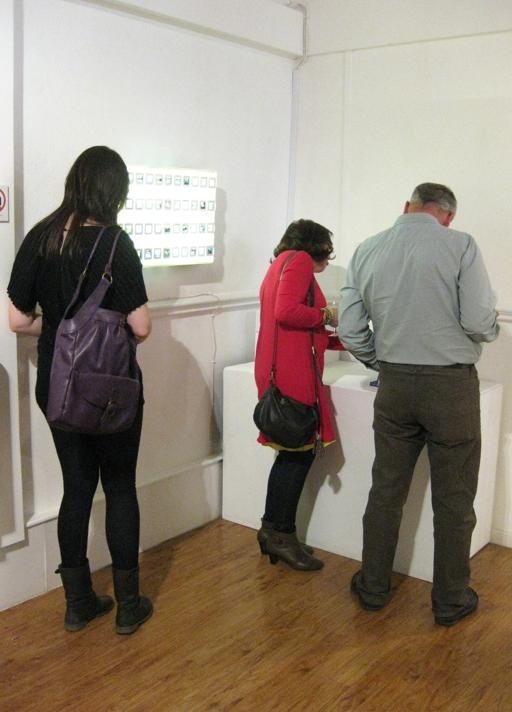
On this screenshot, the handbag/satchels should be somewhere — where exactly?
[47,222,154,436]
[253,386,318,448]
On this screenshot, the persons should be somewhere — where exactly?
[6,147,154,634]
[254,218,347,572]
[336,183,501,628]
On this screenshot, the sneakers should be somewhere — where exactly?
[434,586,479,624]
[352,570,385,611]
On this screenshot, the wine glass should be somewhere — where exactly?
[327,295,339,337]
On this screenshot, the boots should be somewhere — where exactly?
[112,567,153,634]
[56,559,113,632]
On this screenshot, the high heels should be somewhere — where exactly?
[266,529,325,570]
[256,518,314,555]
